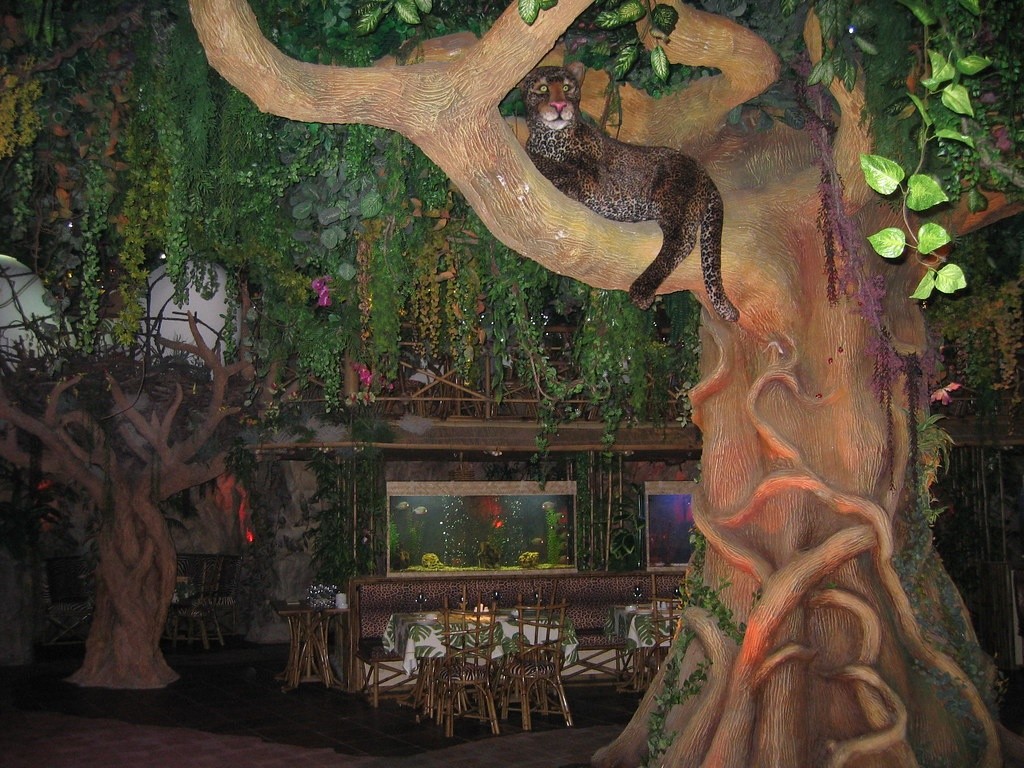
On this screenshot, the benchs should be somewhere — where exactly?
[349,569,685,693]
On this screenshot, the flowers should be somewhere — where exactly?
[307,584,340,609]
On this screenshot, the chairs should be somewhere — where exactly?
[43,553,236,649]
[636,573,681,689]
[413,580,575,736]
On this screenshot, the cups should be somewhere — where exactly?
[335,592,347,608]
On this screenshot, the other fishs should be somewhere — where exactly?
[411,506,427,515]
[541,500,557,511]
[556,531,569,562]
[529,538,543,546]
[393,502,409,511]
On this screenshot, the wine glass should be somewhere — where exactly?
[535,592,541,605]
[459,594,469,611]
[493,589,501,609]
[415,593,426,617]
[633,587,642,605]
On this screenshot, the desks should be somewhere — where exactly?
[604,606,679,689]
[271,599,351,689]
[383,608,578,715]
